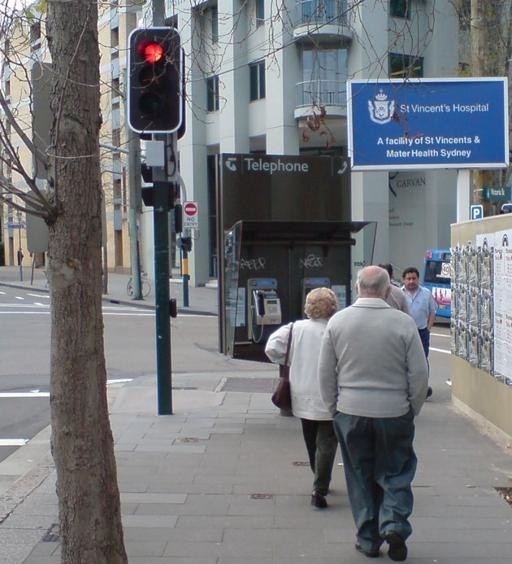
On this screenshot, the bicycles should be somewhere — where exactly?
[127,269,151,297]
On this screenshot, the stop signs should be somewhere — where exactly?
[184,200,198,218]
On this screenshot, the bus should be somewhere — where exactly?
[421,248,451,319]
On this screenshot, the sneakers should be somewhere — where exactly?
[386,531,406,560]
[426,387,432,399]
[355,541,379,557]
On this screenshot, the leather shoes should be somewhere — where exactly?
[312,491,326,508]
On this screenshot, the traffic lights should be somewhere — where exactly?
[126,26,183,137]
[141,160,177,207]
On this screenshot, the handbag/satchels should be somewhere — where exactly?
[272,377,291,410]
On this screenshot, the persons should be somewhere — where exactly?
[263,285,342,509]
[315,263,430,563]
[369,262,411,322]
[392,267,439,400]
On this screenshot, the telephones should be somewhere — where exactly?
[253,290,281,326]
